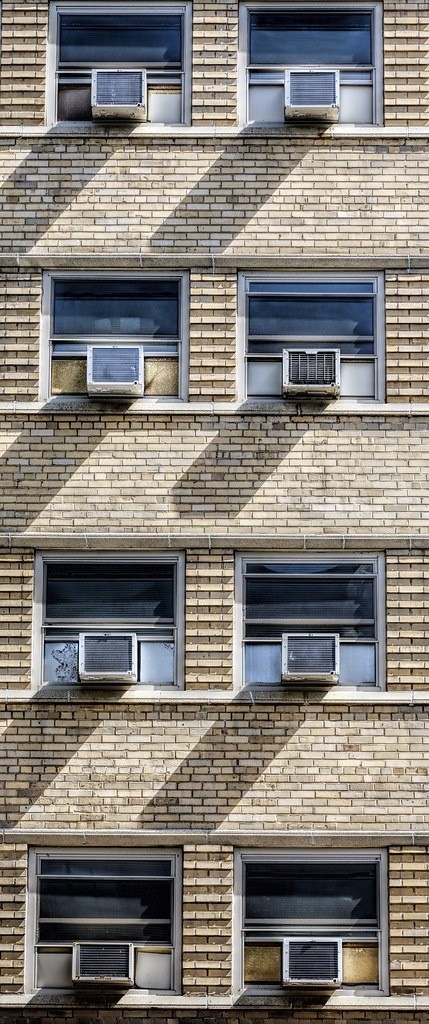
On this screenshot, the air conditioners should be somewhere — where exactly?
[78,631,138,684]
[282,347,341,400]
[283,936,343,989]
[86,344,144,398]
[72,941,134,988]
[91,68,148,123]
[284,69,340,123]
[281,632,340,685]
